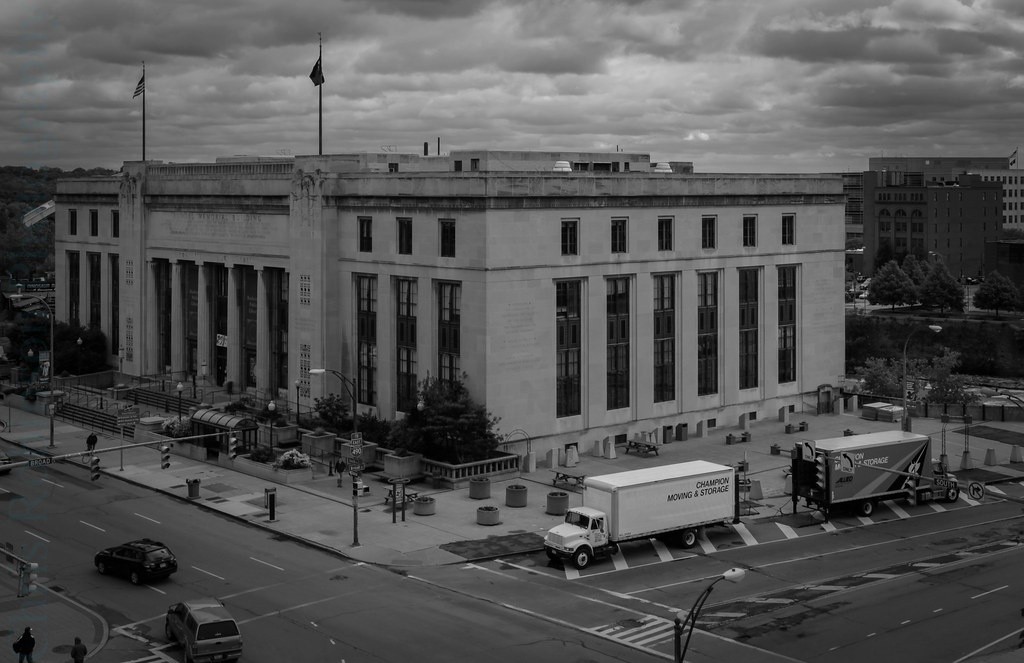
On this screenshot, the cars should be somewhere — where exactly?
[0,449,13,476]
[957,274,985,285]
[857,275,872,290]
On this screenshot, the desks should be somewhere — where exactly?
[550,469,582,487]
[626,438,656,455]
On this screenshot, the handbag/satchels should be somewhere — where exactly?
[12,640,20,654]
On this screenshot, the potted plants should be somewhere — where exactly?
[506,483,528,508]
[383,447,423,481]
[412,494,437,515]
[301,426,337,457]
[264,416,297,443]
[476,504,500,525]
[339,438,378,471]
[469,475,491,499]
[545,490,570,515]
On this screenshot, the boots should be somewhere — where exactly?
[336,479,340,487]
[339,479,343,488]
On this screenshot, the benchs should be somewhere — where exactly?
[735,435,746,439]
[553,476,583,489]
[792,425,804,428]
[624,445,658,458]
[777,448,793,452]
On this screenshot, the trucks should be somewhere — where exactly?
[791,430,959,523]
[543,459,736,570]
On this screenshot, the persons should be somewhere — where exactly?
[12,627,35,663]
[71,637,87,663]
[329,456,346,488]
[86,432,97,456]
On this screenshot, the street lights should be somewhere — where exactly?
[674,565,747,663]
[177,381,183,423]
[307,368,359,546]
[902,324,944,432]
[268,400,275,449]
[295,378,301,424]
[10,294,53,391]
[27,347,35,381]
[77,336,84,374]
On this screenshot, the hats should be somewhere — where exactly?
[24,626,32,632]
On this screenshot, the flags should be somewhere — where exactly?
[308,47,325,87]
[133,70,145,99]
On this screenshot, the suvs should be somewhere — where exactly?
[166,596,243,663]
[95,538,178,586]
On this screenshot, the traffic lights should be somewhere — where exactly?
[21,561,39,596]
[229,436,237,460]
[162,446,171,469]
[352,481,363,497]
[90,455,100,482]
[362,485,370,493]
